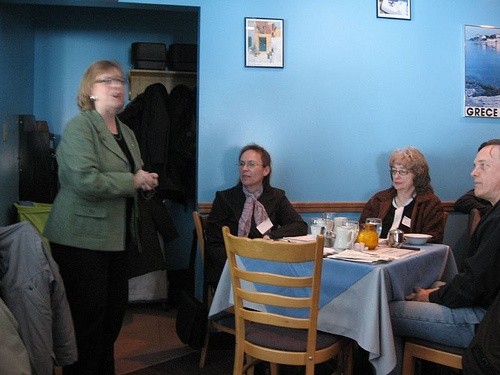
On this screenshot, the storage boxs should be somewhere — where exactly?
[130,42,168,71]
[167,43,198,72]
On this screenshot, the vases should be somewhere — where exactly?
[376,0,411,20]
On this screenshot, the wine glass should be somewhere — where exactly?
[345,221,359,251]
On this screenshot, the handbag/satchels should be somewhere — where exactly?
[177,301,207,350]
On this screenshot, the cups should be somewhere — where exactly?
[333,217,348,227]
[366,218,382,238]
[388,229,404,243]
[324,212,336,219]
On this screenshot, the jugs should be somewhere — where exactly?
[332,226,356,253]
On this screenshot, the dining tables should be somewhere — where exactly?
[209,235,458,375]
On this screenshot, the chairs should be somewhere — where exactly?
[194,209,500,375]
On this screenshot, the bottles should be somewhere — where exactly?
[310,218,336,247]
[356,223,378,250]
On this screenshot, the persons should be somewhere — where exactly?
[388,138,500,375]
[358,149,445,244]
[206,145,308,287]
[43,60,158,375]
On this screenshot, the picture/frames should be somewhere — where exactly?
[244,17,284,68]
[463,24,500,116]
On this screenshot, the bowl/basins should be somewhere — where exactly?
[403,234,433,245]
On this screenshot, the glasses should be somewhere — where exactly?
[92,77,126,85]
[390,170,415,176]
[235,161,265,168]
